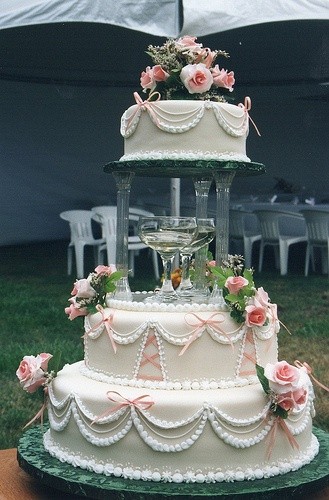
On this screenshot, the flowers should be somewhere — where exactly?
[253,360,310,419]
[140,35,236,104]
[65,265,124,321]
[205,252,270,327]
[16,353,56,394]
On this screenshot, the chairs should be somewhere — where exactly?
[59,200,329,284]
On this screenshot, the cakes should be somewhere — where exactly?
[118,37,260,161]
[18,247,320,484]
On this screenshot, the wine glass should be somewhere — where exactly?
[174,219,216,297]
[137,215,197,303]
